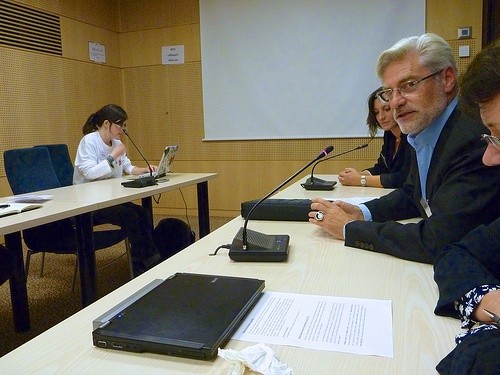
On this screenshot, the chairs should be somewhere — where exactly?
[0,143,135,294]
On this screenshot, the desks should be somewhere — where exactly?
[0,173,222,334]
[0,175,469,375]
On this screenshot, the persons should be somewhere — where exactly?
[73,103,161,278]
[308,33,500,375]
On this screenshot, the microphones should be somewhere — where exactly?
[120,129,157,188]
[304,143,368,191]
[228,145,335,261]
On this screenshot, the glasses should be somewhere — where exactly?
[480,133,500,150]
[376,69,445,104]
[111,121,128,129]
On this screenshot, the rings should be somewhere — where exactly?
[315,211,323,221]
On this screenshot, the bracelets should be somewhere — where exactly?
[106,156,114,165]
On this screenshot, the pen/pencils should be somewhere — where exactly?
[0,205,10,208]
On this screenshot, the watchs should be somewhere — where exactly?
[360,176,366,187]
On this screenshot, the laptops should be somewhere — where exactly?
[92,272,265,360]
[127,145,179,180]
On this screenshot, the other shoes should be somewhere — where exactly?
[132,253,162,274]
